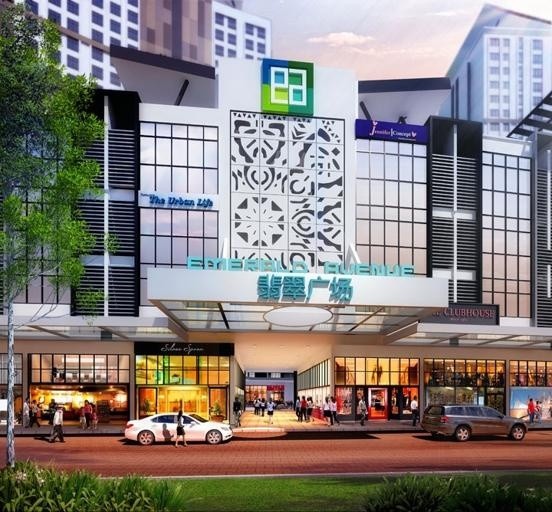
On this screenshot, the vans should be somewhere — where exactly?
[419,403,531,441]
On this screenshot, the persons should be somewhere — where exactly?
[428,370,552,387]
[23,398,98,443]
[527,397,552,426]
[175,409,187,447]
[232,395,420,426]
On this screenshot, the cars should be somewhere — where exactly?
[124,413,236,446]
